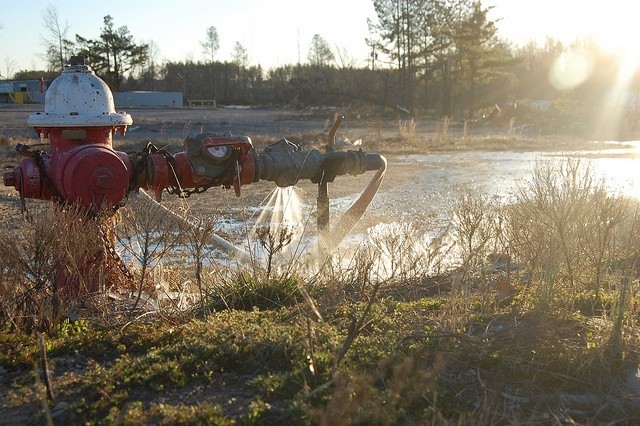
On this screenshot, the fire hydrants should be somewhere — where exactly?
[0,54,388,315]
